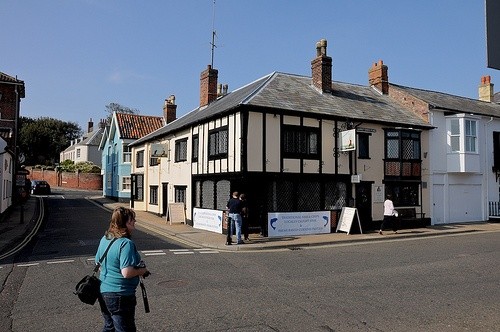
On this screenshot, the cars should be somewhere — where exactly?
[31,181,51,195]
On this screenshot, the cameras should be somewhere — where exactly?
[137,261,151,279]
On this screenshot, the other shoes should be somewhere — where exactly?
[237,242,245,244]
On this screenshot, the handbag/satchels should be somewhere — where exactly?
[75,274,102,305]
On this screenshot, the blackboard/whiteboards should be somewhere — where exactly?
[167,203,185,222]
[337,207,362,232]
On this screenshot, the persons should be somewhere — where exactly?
[95,207,146,332]
[226,192,245,244]
[379,195,397,234]
[239,194,251,241]
